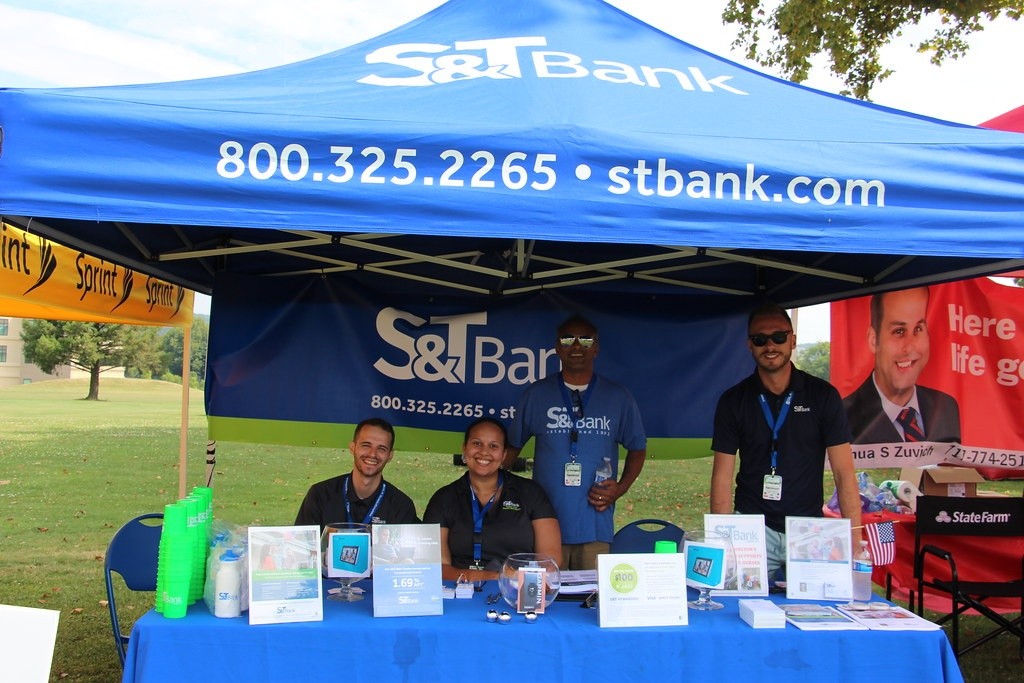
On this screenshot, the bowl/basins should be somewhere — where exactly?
[498,553,561,608]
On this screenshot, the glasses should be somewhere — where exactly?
[748,329,793,347]
[559,333,598,348]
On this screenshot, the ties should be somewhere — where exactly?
[896,407,926,442]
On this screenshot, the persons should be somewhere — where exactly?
[842,283,961,447]
[501,317,646,569]
[293,418,423,580]
[710,306,862,595]
[373,526,414,564]
[423,417,563,592]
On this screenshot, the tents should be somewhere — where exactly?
[0,0,1022,503]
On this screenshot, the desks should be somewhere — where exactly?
[823,504,1024,614]
[123,578,964,683]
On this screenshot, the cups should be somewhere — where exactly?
[154,486,213,618]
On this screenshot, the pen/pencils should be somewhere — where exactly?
[493,593,502,603]
[487,594,493,604]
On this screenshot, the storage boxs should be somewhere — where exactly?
[899,466,986,497]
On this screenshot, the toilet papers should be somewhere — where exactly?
[879,480,924,513]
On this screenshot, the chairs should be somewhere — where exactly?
[105,513,164,669]
[610,519,684,554]
[914,495,1024,663]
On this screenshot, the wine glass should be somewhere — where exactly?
[321,522,372,602]
[679,530,736,610]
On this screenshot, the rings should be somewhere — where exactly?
[598,495,602,500]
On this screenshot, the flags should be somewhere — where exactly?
[864,520,896,566]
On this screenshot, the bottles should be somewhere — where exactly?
[203,526,248,618]
[852,541,873,600]
[593,457,612,489]
[827,472,897,513]
